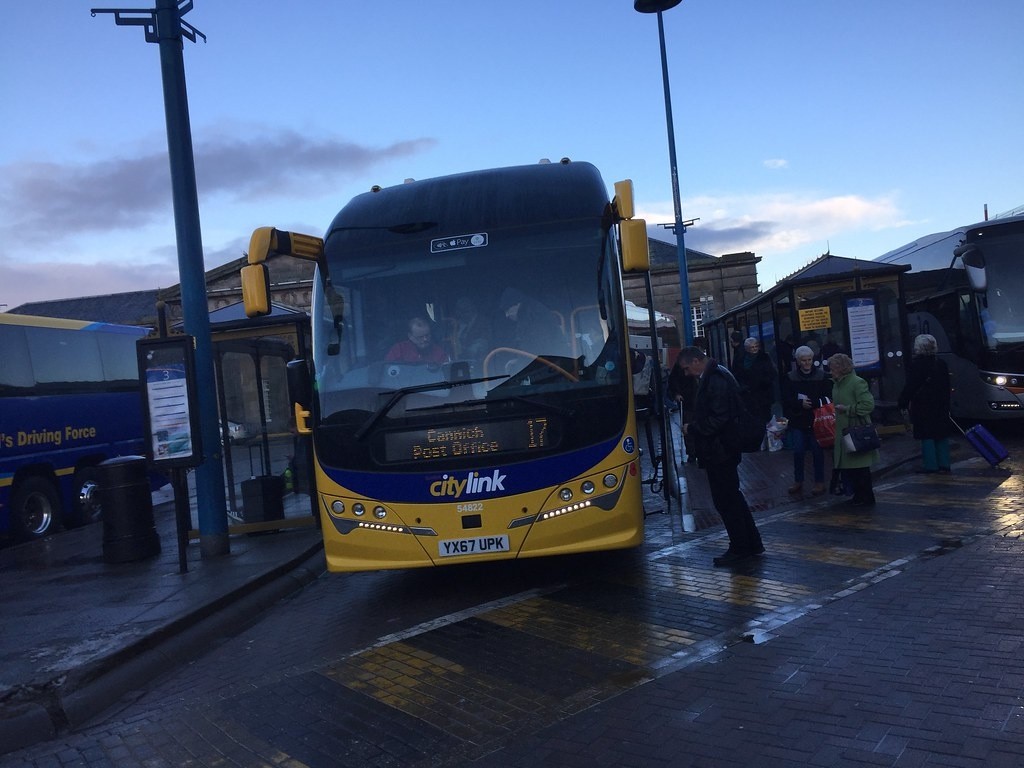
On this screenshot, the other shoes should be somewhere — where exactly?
[915,463,950,472]
[812,483,826,494]
[714,547,761,566]
[846,498,876,508]
[789,480,804,492]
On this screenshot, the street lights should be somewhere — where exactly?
[634,0,698,346]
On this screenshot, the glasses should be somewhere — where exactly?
[409,334,433,342]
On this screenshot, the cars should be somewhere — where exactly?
[218,417,248,446]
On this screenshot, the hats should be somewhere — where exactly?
[499,285,526,311]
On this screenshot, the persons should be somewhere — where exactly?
[667,337,708,468]
[729,331,778,452]
[802,330,823,361]
[387,318,446,363]
[770,334,794,371]
[494,287,562,371]
[827,353,880,514]
[898,334,952,474]
[678,347,764,576]
[783,346,834,496]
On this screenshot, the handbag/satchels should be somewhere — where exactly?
[843,410,882,456]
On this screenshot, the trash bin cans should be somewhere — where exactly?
[97,455,162,565]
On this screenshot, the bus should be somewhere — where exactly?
[239,157,650,574]
[625,300,682,413]
[867,212,1024,423]
[0,312,170,542]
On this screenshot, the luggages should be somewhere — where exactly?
[947,411,1009,470]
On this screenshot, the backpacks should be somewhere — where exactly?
[813,396,837,447]
